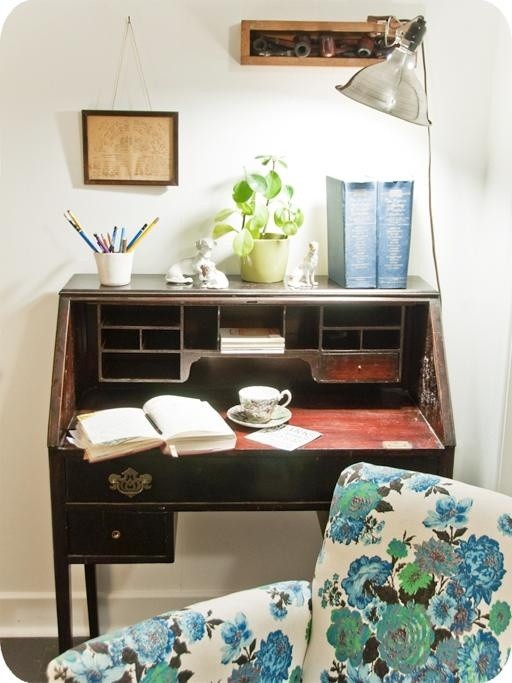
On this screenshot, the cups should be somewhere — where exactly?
[238,385,292,423]
[92,251,135,286]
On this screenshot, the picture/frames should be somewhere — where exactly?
[81,109,179,187]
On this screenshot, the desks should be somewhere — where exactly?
[45,272,457,654]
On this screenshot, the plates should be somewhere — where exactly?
[227,404,292,430]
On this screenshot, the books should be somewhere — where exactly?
[326,173,415,290]
[219,327,286,354]
[66,395,236,464]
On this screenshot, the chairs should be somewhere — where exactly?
[45,462,512,683]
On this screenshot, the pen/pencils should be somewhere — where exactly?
[63,208,160,253]
[145,414,162,435]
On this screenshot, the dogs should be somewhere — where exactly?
[199,263,230,290]
[164,237,218,284]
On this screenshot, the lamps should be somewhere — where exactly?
[335,13,433,129]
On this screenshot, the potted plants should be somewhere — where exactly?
[212,153,305,284]
